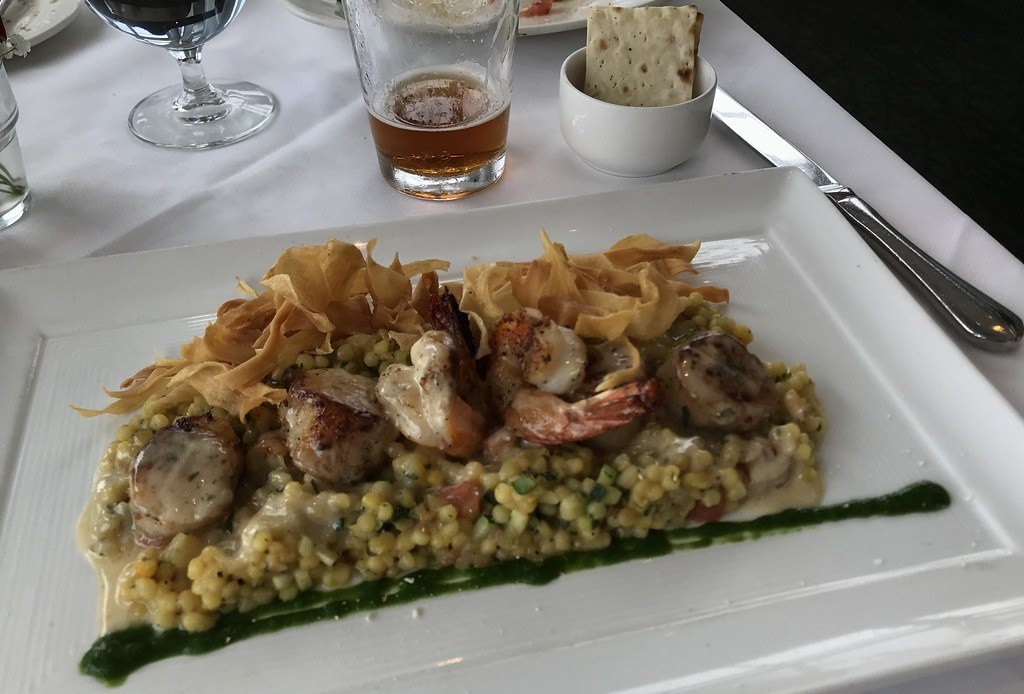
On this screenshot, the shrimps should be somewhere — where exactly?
[380,313,662,454]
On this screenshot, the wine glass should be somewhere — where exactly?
[81,2,275,151]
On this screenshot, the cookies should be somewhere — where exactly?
[581,5,704,108]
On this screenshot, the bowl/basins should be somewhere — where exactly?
[556,45,718,181]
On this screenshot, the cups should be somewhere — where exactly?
[1,61,32,232]
[335,0,516,206]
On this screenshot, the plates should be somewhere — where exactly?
[286,0,674,37]
[2,2,82,53]
[0,162,1024,694]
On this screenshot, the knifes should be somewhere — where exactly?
[711,85,1024,351]
